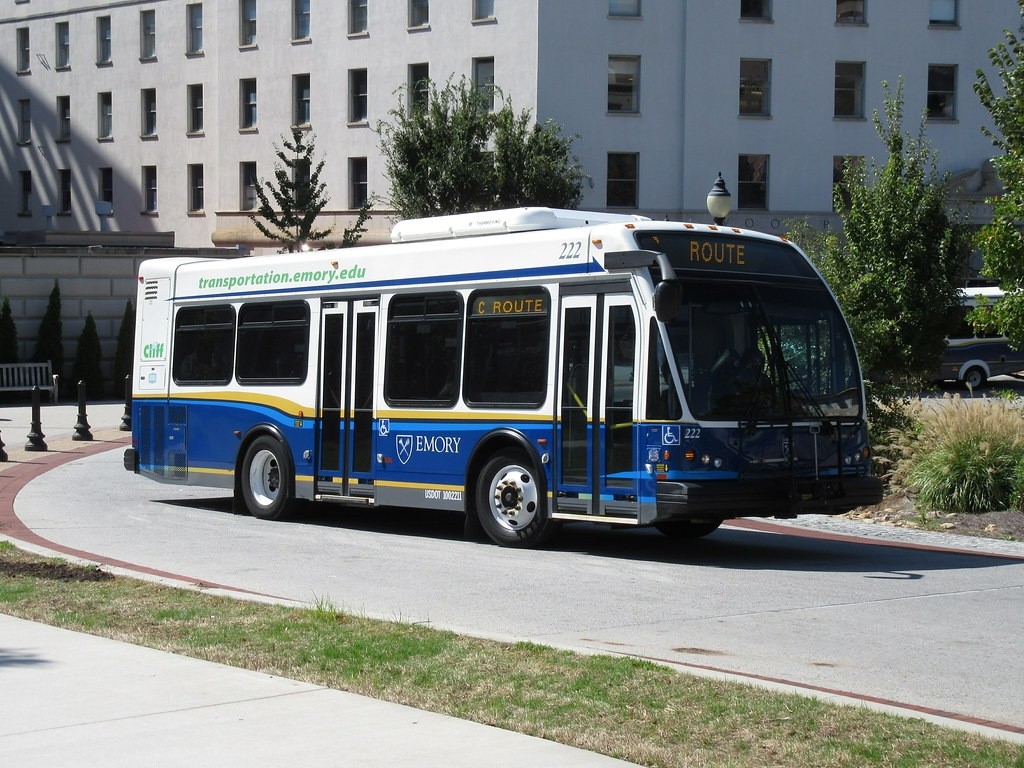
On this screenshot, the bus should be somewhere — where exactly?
[121,208,884,554]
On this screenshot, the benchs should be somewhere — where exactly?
[0,359,59,406]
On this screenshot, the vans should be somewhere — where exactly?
[922,286,1023,388]
